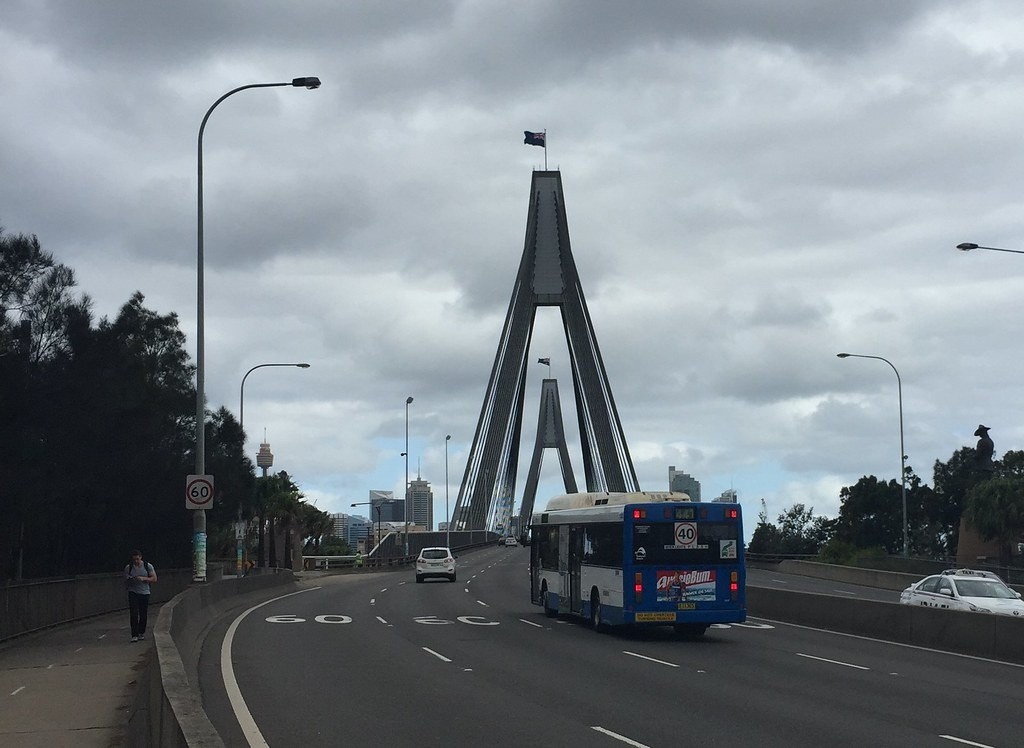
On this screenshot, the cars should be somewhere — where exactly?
[523,538,532,547]
[498,538,506,546]
[505,537,517,548]
[900,568,1024,617]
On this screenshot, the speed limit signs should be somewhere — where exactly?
[184,474,214,510]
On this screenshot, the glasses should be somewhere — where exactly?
[133,558,139,560]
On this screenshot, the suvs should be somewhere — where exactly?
[416,547,458,583]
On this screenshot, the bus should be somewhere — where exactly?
[520,492,747,638]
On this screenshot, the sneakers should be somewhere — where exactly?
[130,634,144,643]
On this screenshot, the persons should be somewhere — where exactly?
[355,550,363,568]
[243,558,254,576]
[124,550,157,643]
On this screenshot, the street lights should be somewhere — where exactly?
[402,397,413,556]
[366,527,371,568]
[484,468,489,541]
[956,242,1023,254]
[194,77,323,582]
[236,363,311,580]
[838,353,912,557]
[445,435,450,548]
[351,503,381,567]
